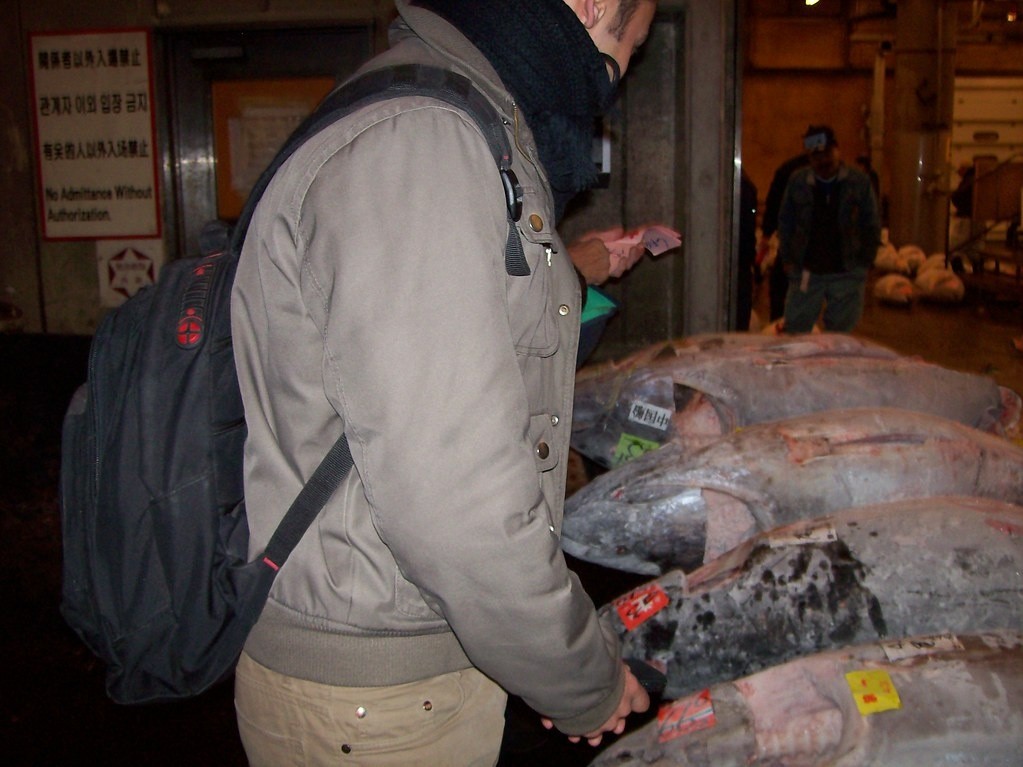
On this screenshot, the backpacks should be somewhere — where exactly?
[56,59,528,718]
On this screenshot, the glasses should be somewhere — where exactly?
[809,152,834,161]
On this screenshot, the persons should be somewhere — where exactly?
[734,124,886,334]
[221,0,659,767]
[948,157,991,277]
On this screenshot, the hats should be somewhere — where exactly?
[802,125,836,155]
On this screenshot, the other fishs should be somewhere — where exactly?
[580,629,1023,767]
[591,496,1023,698]
[574,327,1022,471]
[870,241,967,310]
[555,407,1023,577]
